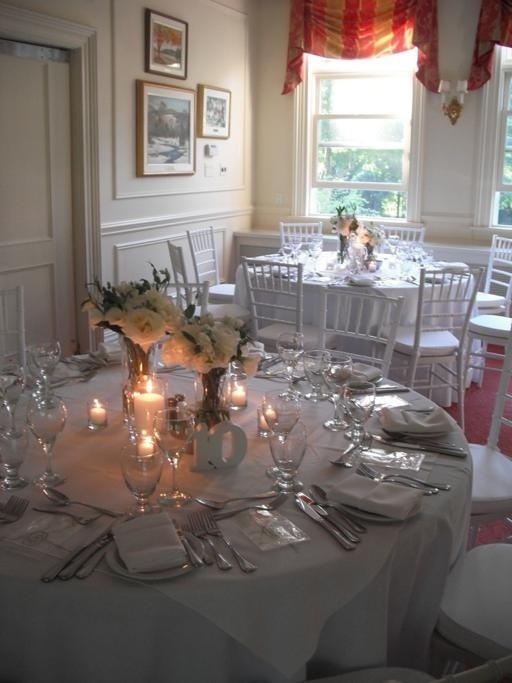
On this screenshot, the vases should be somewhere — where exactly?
[192,369,233,430]
[337,240,352,264]
[362,242,382,273]
[120,338,157,424]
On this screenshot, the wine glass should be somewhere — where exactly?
[30,338,63,407]
[277,331,304,400]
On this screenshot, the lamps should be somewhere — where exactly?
[439,78,470,124]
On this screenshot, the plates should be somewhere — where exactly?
[0,349,467,584]
[247,231,470,283]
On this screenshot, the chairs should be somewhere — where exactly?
[316,655,511,682]
[380,224,426,255]
[185,226,236,304]
[468,325,512,527]
[166,241,249,327]
[437,543,512,662]
[278,219,323,255]
[463,234,512,391]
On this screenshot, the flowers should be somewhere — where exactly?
[83,262,183,413]
[331,203,359,239]
[356,221,384,249]
[161,311,256,425]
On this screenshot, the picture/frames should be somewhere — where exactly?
[134,78,198,176]
[196,83,232,139]
[144,7,189,79]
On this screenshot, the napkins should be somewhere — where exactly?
[376,405,454,436]
[333,471,424,521]
[110,512,189,575]
[335,361,383,382]
